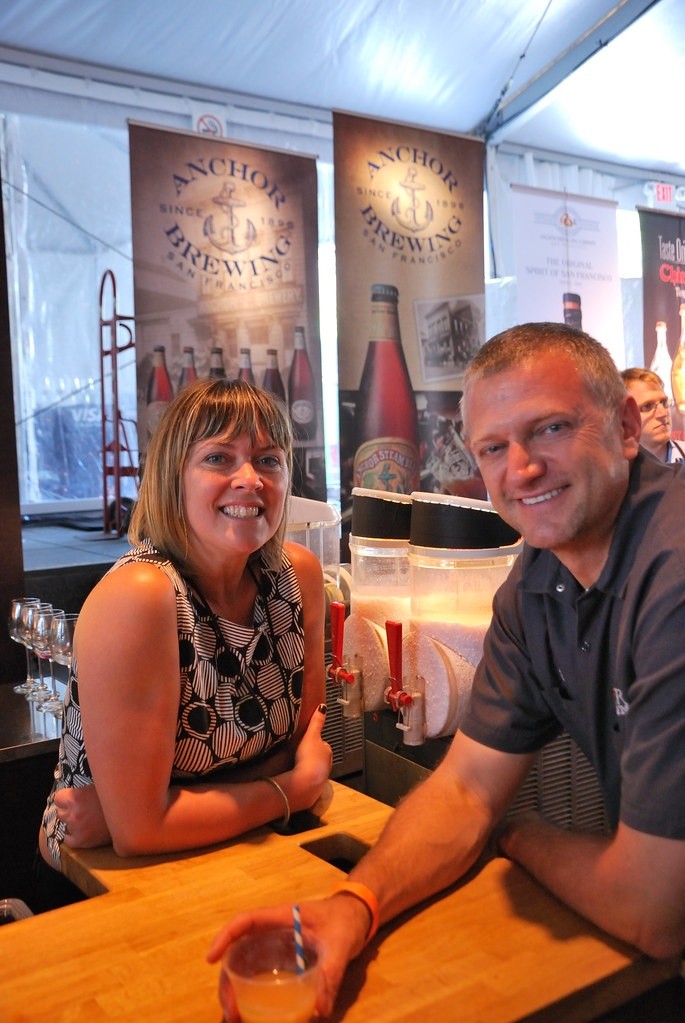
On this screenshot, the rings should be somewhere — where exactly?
[63,823,69,834]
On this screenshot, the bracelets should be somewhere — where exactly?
[266,778,290,830]
[327,883,381,943]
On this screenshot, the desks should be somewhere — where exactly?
[0,779,655,1023]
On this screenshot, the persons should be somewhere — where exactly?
[205,323,685,1023]
[621,369,685,464]
[31,379,332,915]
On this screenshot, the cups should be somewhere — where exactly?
[222,928,326,1023]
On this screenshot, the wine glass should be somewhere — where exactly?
[8,597,80,719]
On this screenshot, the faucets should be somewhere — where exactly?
[324,601,362,720]
[385,620,425,747]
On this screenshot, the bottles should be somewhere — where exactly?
[650,321,674,407]
[144,345,173,442]
[352,283,420,495]
[237,348,255,384]
[262,349,285,403]
[563,293,583,331]
[670,303,685,416]
[208,347,226,378]
[178,346,197,391]
[288,326,317,441]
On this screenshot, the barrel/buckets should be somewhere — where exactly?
[283,488,524,746]
